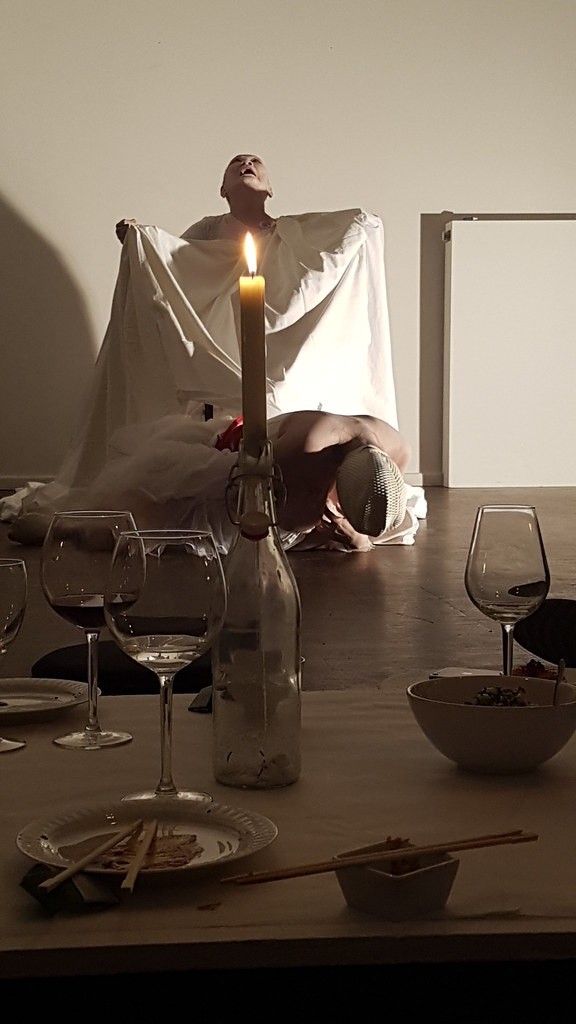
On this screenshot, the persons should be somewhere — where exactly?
[83,408,408,555]
[113,153,386,248]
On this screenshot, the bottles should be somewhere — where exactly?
[210,437,306,789]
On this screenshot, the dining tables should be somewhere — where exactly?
[0,692,575,976]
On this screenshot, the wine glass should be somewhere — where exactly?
[0,557,31,756]
[463,504,552,676]
[99,526,228,808]
[38,509,145,752]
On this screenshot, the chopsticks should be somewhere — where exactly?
[120,819,161,897]
[37,818,144,893]
[219,826,541,887]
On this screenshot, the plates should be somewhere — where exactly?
[14,796,277,880]
[0,677,101,725]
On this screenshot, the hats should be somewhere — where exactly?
[337,446,408,539]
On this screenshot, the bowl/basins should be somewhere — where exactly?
[329,840,462,920]
[407,674,576,779]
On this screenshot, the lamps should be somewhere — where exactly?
[236,230,269,458]
